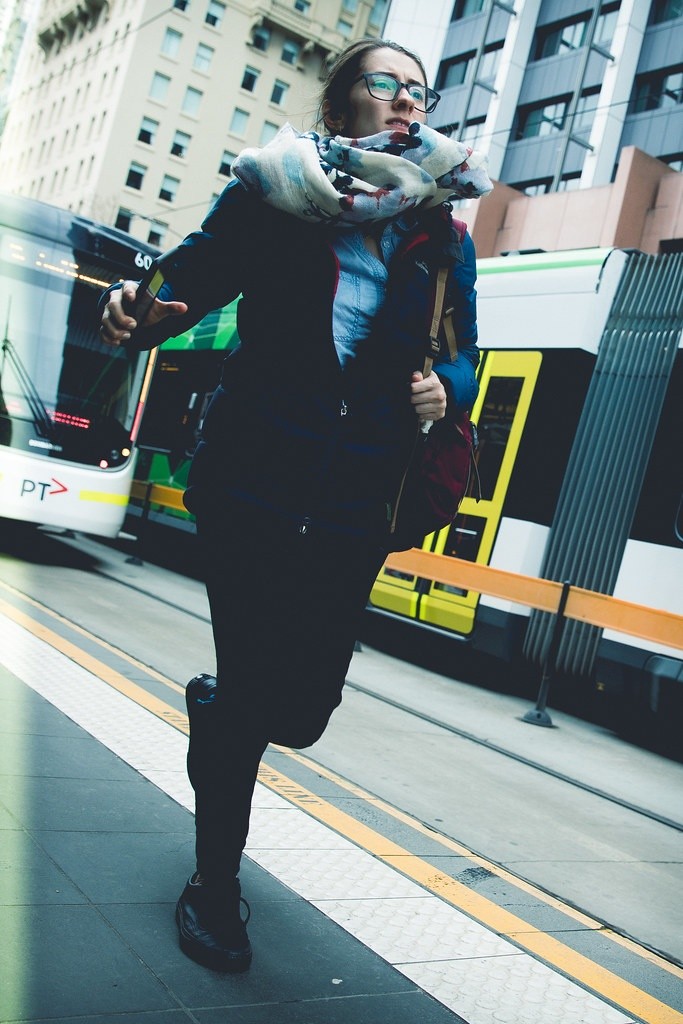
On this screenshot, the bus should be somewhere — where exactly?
[0,186,683,730]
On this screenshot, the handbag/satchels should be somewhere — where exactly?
[393,410,480,552]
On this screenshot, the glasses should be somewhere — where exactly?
[350,72,441,113]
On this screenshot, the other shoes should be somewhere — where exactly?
[175,870,253,972]
[186,672,217,791]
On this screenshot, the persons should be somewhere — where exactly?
[95,40,482,976]
